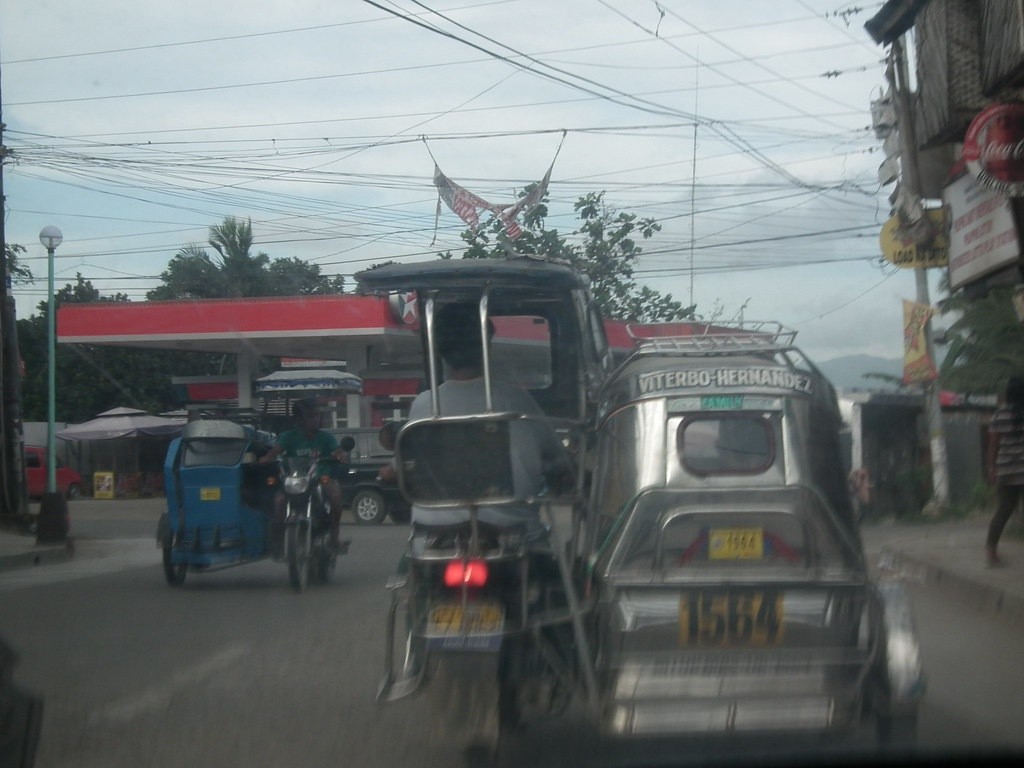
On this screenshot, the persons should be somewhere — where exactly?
[986,376,1024,562]
[264,400,350,555]
[857,470,880,524]
[392,302,574,677]
[717,418,773,474]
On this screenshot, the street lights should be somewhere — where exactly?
[37,228,74,547]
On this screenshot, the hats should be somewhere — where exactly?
[436,301,495,347]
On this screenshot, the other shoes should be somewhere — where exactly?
[328,543,350,556]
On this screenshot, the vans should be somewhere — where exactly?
[24,445,84,501]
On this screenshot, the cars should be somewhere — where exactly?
[156,370,355,592]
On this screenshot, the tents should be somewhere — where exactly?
[21,421,89,464]
[55,405,188,497]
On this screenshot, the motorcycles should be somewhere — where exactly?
[354,258,923,768]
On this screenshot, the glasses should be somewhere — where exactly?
[300,412,321,418]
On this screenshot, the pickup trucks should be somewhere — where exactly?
[337,452,410,524]
